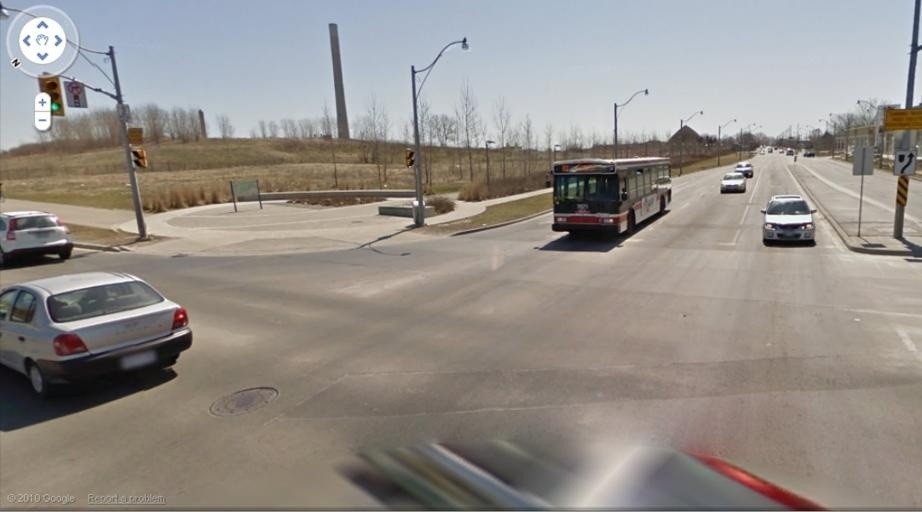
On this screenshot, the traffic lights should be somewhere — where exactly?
[406,148,415,168]
[38,77,64,116]
[130,148,148,170]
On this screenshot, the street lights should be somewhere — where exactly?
[486,141,495,199]
[411,38,469,225]
[718,119,762,167]
[554,145,561,161]
[679,111,704,174]
[0,4,147,237]
[818,100,884,161]
[614,89,648,158]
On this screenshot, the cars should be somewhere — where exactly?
[760,195,817,246]
[734,160,753,178]
[720,172,747,193]
[0,210,72,271]
[0,271,192,399]
[760,144,794,155]
[804,151,815,157]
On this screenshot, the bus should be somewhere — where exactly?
[551,156,672,237]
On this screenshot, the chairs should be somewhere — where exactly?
[59,293,138,317]
